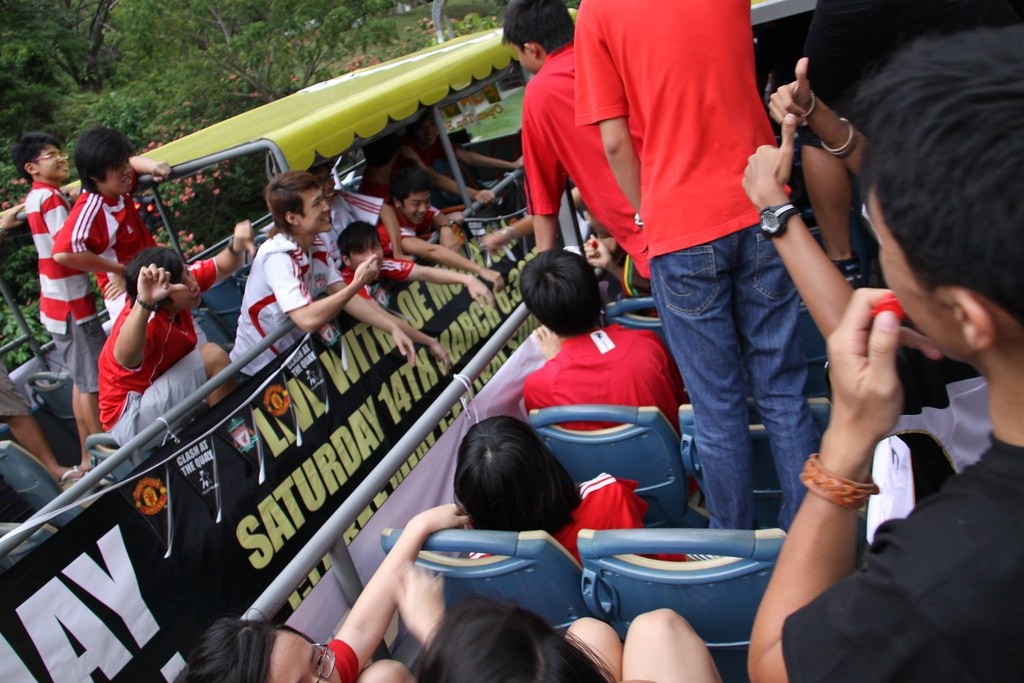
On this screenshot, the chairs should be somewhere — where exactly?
[0,440,109,528]
[0,522,59,572]
[528,403,688,521]
[191,234,269,345]
[677,398,837,522]
[798,209,869,395]
[576,525,792,683]
[605,295,668,350]
[375,522,583,644]
[26,371,84,434]
[84,432,152,483]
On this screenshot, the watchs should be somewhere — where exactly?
[228,237,238,256]
[758,203,797,239]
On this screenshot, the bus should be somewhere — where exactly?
[0,8,579,683]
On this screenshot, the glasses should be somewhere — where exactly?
[315,644,336,683]
[28,152,69,163]
[315,173,334,186]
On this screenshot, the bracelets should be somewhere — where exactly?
[137,296,155,311]
[798,454,878,509]
[440,224,452,228]
[799,90,816,119]
[634,213,644,227]
[500,227,516,239]
[821,118,857,159]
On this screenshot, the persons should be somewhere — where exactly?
[184,0,1024,683]
[0,110,523,485]
